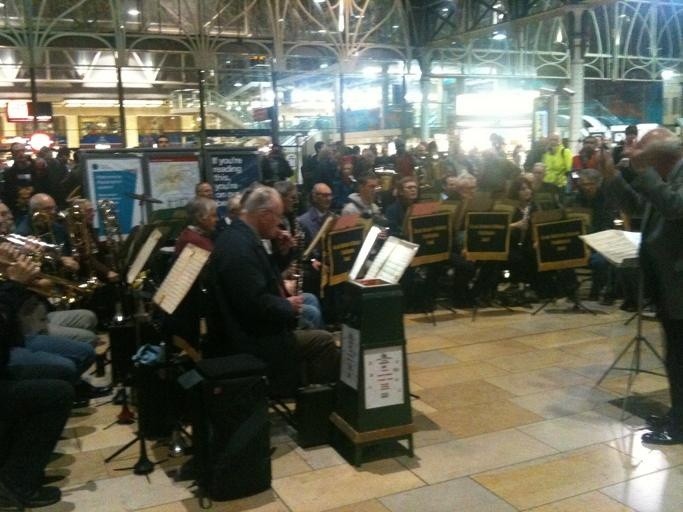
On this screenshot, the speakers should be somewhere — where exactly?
[191,377,271,501]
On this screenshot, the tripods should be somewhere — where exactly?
[597,264,668,421]
[461,261,514,322]
[413,265,457,323]
[532,270,597,317]
[88,234,168,483]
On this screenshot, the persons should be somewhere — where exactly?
[590,128,683,447]
[2,116,652,349]
[0,242,96,437]
[206,186,342,382]
[0,363,76,508]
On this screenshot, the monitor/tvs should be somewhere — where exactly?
[27,102,52,116]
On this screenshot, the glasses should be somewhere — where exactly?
[315,192,331,198]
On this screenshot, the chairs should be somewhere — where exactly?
[198,279,313,434]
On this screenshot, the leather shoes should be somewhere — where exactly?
[643,432,676,445]
[0,486,60,507]
[112,389,126,404]
[88,385,112,398]
[71,399,88,408]
[645,414,672,428]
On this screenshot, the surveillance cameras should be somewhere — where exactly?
[562,88,575,96]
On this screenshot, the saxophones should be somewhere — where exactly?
[0,197,126,309]
